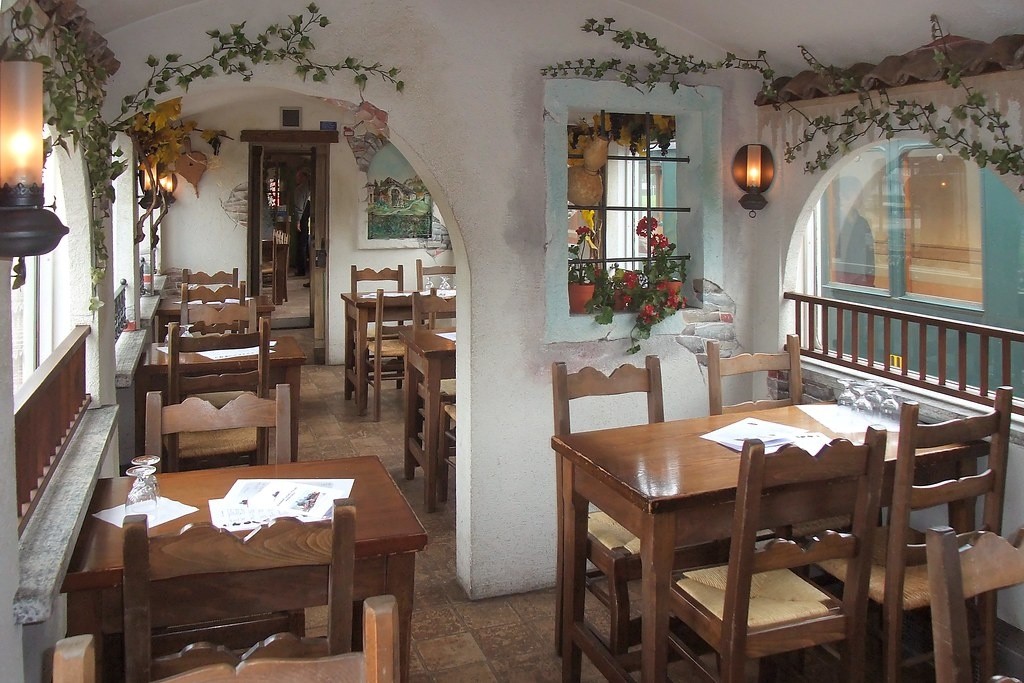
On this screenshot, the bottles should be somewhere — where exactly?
[143,261,150,291]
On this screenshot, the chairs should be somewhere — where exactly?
[416,258,457,329]
[181,299,257,336]
[350,265,406,404]
[145,383,292,475]
[667,424,888,683]
[262,228,290,305]
[182,267,239,288]
[706,335,884,683]
[167,316,270,472]
[437,400,456,504]
[181,281,246,336]
[789,385,1013,683]
[53,595,400,683]
[411,291,457,490]
[924,527,1024,683]
[122,497,357,683]
[364,286,438,422]
[551,355,732,683]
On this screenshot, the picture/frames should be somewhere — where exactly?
[356,138,443,249]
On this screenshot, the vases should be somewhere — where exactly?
[568,135,609,207]
[603,288,633,312]
[654,281,683,308]
[568,281,594,314]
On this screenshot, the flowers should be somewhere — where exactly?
[635,216,688,285]
[567,226,597,285]
[585,270,689,354]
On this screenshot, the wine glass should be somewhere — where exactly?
[164,325,194,351]
[836,378,901,431]
[175,282,182,302]
[424,275,456,296]
[126,454,160,521]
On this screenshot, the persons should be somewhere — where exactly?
[830,178,876,287]
[292,170,312,287]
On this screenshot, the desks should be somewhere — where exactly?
[59,454,429,683]
[398,326,456,514]
[134,336,306,476]
[340,290,457,416]
[155,296,275,343]
[551,402,991,683]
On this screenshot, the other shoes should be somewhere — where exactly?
[303,282,310,287]
[295,272,305,276]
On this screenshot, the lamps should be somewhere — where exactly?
[137,152,163,210]
[0,60,69,258]
[156,167,178,203]
[733,143,775,219]
